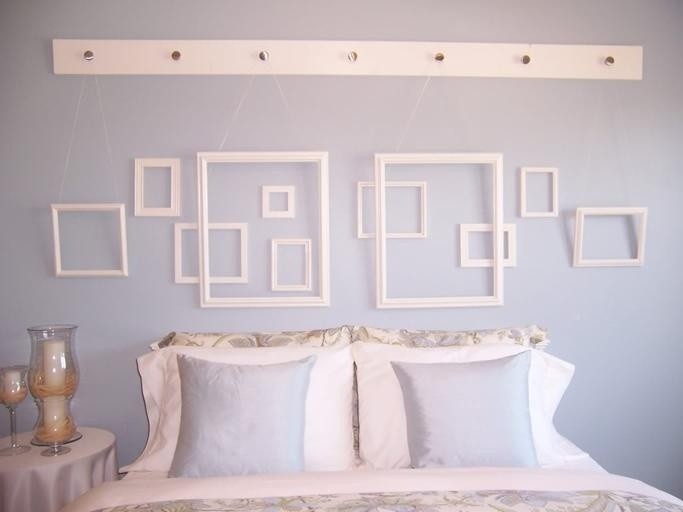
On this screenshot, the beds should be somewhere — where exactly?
[60,320,683,512]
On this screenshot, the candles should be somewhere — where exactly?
[1,319,85,459]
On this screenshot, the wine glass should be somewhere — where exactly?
[33,394,76,456]
[0,364,33,459]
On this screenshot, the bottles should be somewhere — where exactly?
[26,325,84,445]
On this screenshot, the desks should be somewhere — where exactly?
[0,425,118,511]
